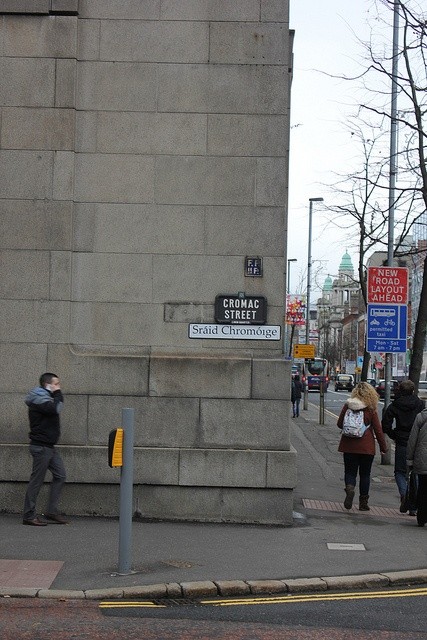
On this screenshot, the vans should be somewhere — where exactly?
[334,373,353,392]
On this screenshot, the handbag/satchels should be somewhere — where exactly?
[404,465,418,510]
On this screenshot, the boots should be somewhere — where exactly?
[343,484,355,509]
[358,495,369,511]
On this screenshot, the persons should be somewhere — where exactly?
[407,406,427,528]
[292,375,303,417]
[23,373,69,526]
[336,381,388,511]
[382,379,424,517]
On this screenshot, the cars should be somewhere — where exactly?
[366,377,377,390]
[414,380,427,399]
[376,378,399,399]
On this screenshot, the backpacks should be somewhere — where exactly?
[340,409,372,439]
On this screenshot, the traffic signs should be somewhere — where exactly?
[367,266,408,303]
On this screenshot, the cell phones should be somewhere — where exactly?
[46,384,52,391]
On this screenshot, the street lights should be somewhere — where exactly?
[303,196,326,410]
[285,258,298,358]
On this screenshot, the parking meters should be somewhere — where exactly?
[106,406,137,574]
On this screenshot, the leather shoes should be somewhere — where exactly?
[399,495,408,513]
[44,511,67,523]
[409,510,416,516]
[22,517,48,526]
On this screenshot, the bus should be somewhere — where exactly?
[291,365,300,378]
[305,356,329,393]
